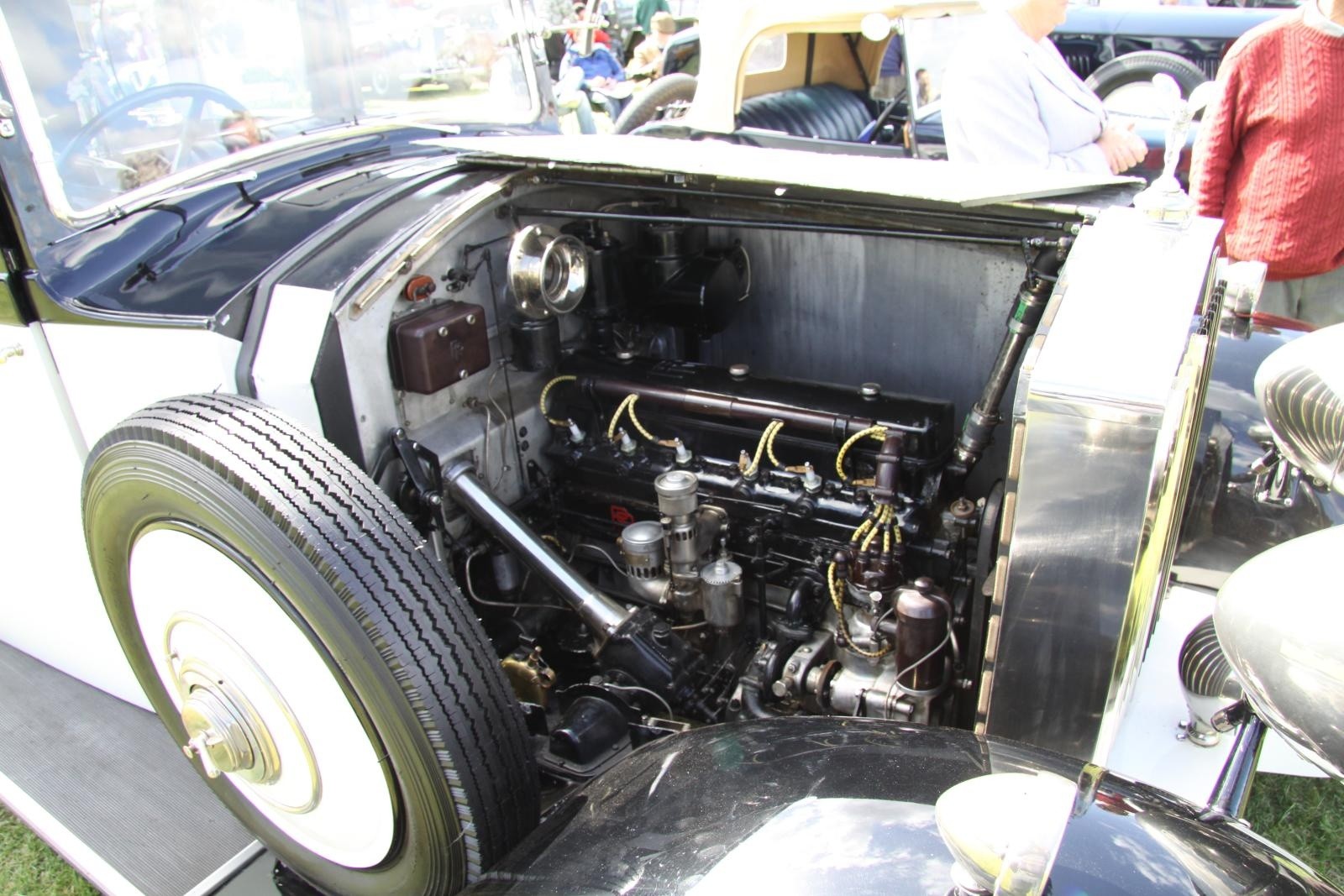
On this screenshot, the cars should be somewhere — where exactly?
[2,1,1344,896]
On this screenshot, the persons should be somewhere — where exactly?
[1188,0,1344,330]
[940,0,1148,180]
[558,0,676,135]
[871,34,907,101]
[916,68,940,107]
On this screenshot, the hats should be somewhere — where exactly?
[651,11,676,34]
